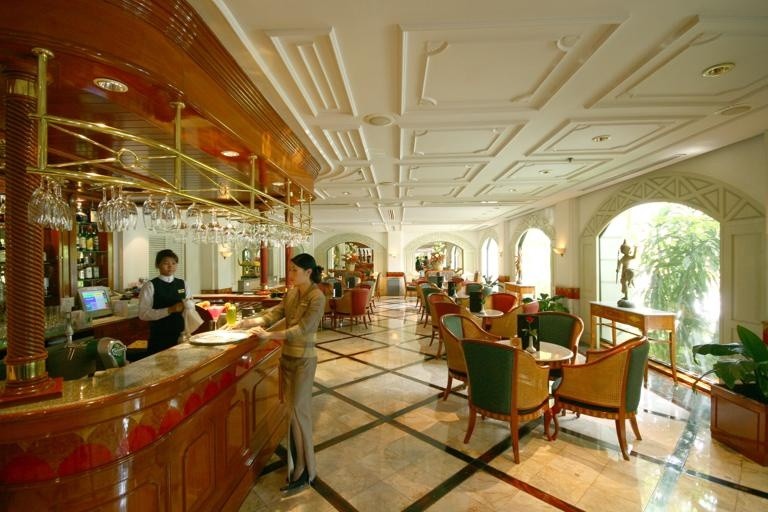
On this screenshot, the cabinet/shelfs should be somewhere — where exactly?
[74,196,110,282]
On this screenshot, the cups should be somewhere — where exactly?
[224,303,237,329]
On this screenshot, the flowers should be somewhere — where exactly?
[430,241,446,264]
[343,251,360,265]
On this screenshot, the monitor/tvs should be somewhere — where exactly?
[77,286,114,318]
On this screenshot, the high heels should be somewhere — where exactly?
[288,465,307,489]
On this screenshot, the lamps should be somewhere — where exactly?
[552,247,566,256]
[219,251,232,260]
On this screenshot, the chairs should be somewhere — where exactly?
[318,272,381,330]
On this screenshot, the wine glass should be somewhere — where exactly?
[25,175,309,248]
[206,306,225,340]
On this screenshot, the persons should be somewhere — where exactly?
[616,238,638,299]
[138,249,195,357]
[513,250,523,280]
[231,252,327,490]
[415,254,429,271]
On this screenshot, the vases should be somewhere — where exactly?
[431,261,441,270]
[345,261,356,271]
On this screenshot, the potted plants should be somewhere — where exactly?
[689,317,768,466]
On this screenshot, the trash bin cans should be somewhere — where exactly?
[387,277,405,296]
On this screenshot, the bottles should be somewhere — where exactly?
[77,248,101,280]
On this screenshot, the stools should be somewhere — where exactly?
[126,339,148,364]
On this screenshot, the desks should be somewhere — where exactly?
[585,299,677,388]
